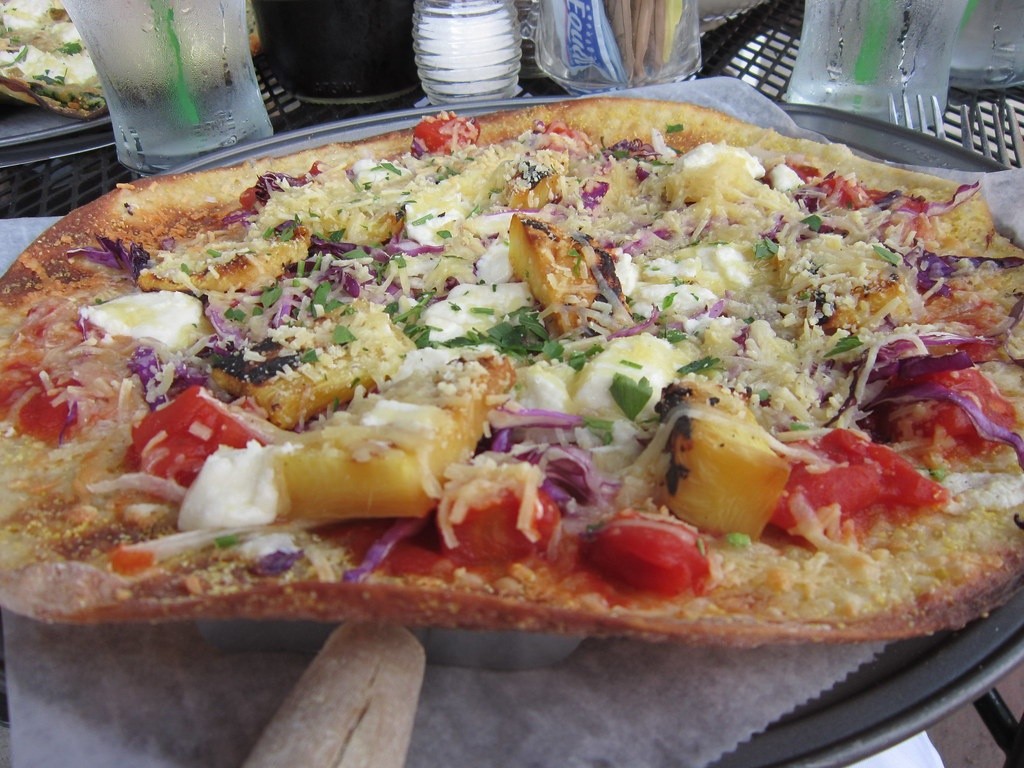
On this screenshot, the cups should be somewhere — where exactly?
[783,0,964,128]
[949,0,1024,92]
[63,0,274,175]
[535,0,702,92]
[412,0,522,106]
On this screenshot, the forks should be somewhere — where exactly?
[887,90,1024,169]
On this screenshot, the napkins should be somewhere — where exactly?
[848,731,947,766]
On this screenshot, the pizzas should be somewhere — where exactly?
[0,100,1024,643]
[1,0,259,120]
